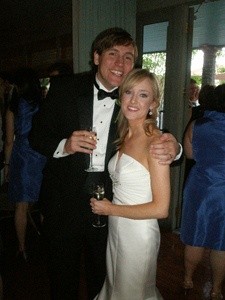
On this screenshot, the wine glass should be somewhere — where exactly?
[91,183,106,228]
[85,127,98,172]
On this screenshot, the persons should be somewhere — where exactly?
[33,27,185,300]
[0,67,225,300]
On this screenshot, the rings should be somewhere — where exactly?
[167,154,173,160]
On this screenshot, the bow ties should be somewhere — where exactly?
[94,81,120,100]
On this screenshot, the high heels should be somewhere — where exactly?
[182,281,194,297]
[211,292,222,300]
